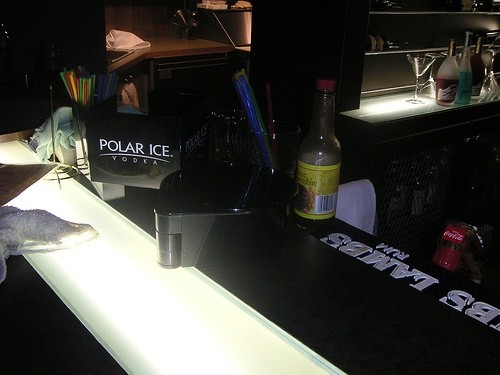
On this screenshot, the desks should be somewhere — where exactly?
[137,36,230,93]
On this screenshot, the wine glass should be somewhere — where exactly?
[404,53,444,105]
[478,48,500,102]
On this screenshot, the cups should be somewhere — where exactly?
[248,120,300,205]
[210,106,252,163]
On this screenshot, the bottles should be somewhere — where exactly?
[470,37,485,96]
[454,32,473,105]
[435,38,460,106]
[292,79,341,233]
